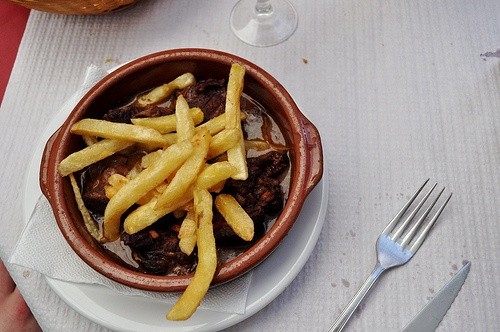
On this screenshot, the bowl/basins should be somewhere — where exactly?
[37,49,327,293]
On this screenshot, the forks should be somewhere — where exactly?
[328,177,456,331]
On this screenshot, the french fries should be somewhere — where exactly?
[59,61,254,320]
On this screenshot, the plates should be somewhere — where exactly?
[20,57,329,331]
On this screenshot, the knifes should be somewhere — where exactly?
[404,260,475,332]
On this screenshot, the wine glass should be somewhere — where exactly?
[230,1,300,47]
